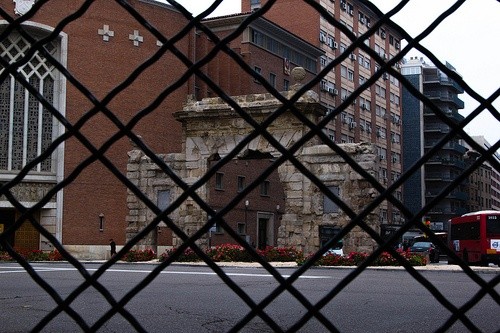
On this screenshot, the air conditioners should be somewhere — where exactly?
[382,33,386,38]
[381,154,384,160]
[352,54,356,61]
[350,122,355,128]
[343,118,348,124]
[398,119,402,124]
[397,64,401,68]
[361,18,365,23]
[395,42,399,48]
[377,131,382,137]
[363,103,368,109]
[368,23,371,28]
[352,99,356,105]
[393,157,397,163]
[350,10,354,15]
[322,84,329,91]
[334,42,338,49]
[333,89,338,95]
[385,54,388,59]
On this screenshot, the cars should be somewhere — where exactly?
[409,242,440,263]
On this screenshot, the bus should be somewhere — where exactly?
[449,210,500,267]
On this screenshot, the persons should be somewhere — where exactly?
[109,239,117,257]
